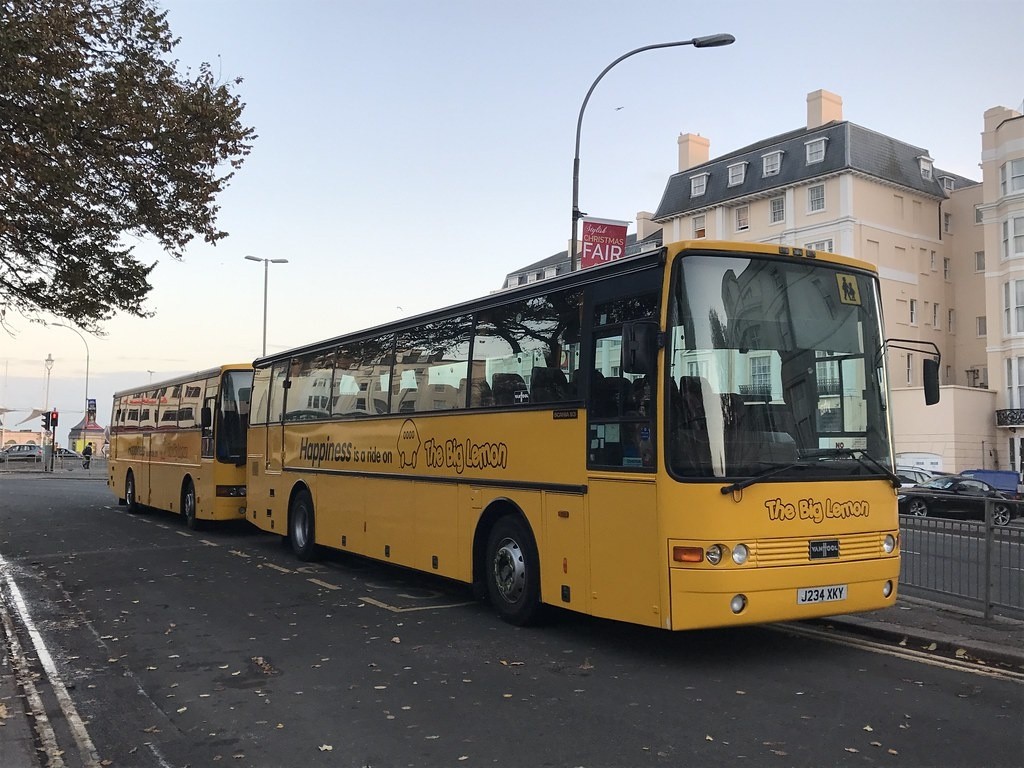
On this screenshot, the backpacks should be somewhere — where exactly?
[82,449,87,455]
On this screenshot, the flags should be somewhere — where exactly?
[87,399,96,423]
[581,215,629,269]
[560,349,568,370]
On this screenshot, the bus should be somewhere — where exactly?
[243,239,944,633]
[108,361,256,530]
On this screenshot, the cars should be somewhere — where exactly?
[0,444,42,462]
[54,447,77,456]
[898,466,1023,525]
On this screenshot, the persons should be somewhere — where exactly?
[82,442,92,469]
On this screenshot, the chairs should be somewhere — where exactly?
[335,370,746,429]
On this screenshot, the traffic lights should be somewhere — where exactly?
[41,411,50,429]
[52,411,59,426]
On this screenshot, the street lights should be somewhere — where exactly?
[568,30,736,269]
[146,369,156,384]
[44,353,55,410]
[52,321,89,452]
[245,255,289,357]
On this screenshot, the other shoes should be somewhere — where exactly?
[83,465,86,469]
[87,467,89,469]
